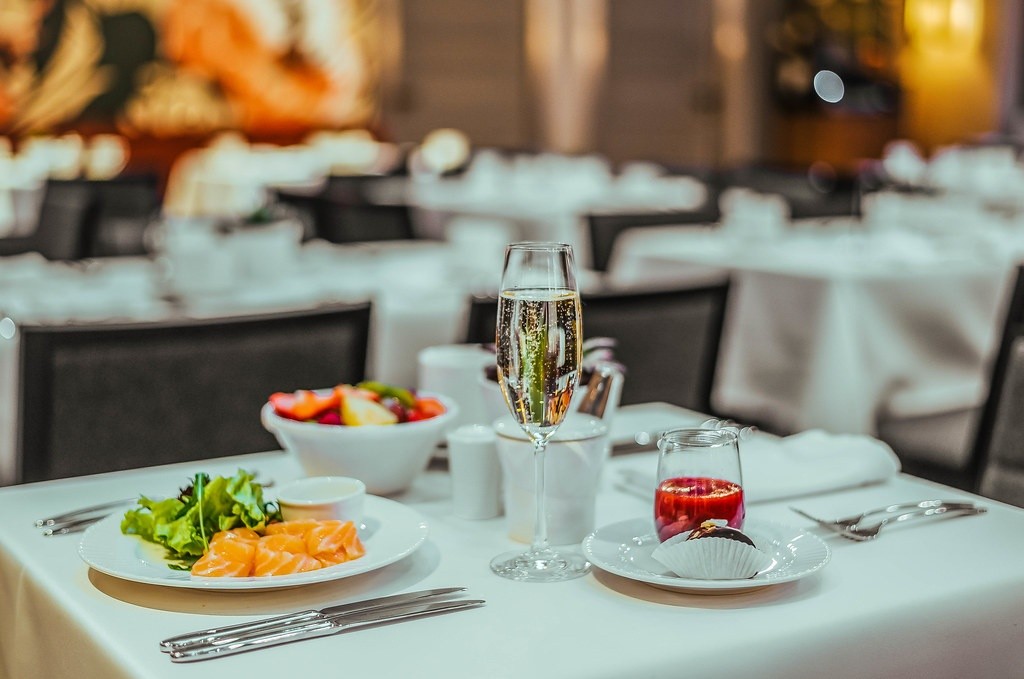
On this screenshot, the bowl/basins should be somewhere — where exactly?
[261,388,459,494]
[278,477,367,527]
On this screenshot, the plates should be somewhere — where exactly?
[81,487,430,592]
[583,515,831,596]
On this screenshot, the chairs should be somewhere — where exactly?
[462,271,734,409]
[15,300,373,486]
[955,264,1024,507]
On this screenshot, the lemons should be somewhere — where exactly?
[340,392,398,426]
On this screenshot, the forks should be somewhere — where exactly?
[789,495,988,544]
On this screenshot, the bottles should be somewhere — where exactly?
[444,424,501,521]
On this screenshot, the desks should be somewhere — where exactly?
[0,238,601,487]
[608,216,1024,464]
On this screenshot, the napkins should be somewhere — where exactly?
[612,427,902,503]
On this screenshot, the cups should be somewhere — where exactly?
[654,430,747,545]
[414,343,498,448]
[495,415,609,546]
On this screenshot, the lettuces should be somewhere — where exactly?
[120,467,282,570]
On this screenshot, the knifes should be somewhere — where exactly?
[155,585,486,663]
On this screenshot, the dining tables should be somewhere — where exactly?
[0,401,1024,679]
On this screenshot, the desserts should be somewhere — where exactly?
[651,522,765,579]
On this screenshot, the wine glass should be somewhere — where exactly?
[493,239,592,584]
